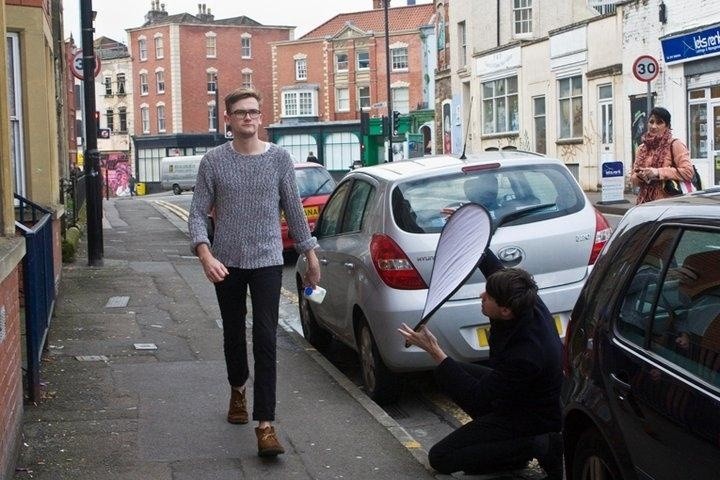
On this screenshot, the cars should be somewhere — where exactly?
[294,95,613,400]
[207,161,337,253]
[556,187,720,479]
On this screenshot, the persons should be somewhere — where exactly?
[184,86,324,457]
[398,202,566,475]
[307,149,318,163]
[631,106,695,202]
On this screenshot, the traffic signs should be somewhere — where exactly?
[632,55,659,82]
[69,49,101,81]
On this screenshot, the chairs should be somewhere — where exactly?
[659,248,719,368]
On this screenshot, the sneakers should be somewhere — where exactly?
[255,426,285,458]
[227,387,249,425]
[536,433,563,479]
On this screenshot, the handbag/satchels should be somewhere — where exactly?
[664,167,701,197]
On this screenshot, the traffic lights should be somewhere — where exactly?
[392,109,400,133]
[380,114,388,136]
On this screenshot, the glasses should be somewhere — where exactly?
[229,109,261,120]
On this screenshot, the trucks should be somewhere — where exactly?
[159,155,204,195]
